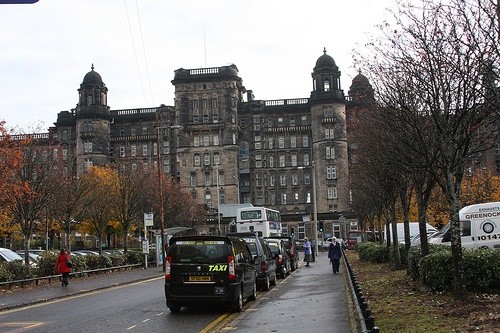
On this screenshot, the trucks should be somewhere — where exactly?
[384,221,438,244]
[427,201,500,251]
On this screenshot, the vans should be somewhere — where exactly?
[164,232,300,313]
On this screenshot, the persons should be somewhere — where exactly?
[328,237,342,274]
[56,249,72,287]
[301,237,311,266]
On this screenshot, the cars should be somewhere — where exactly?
[324,237,344,248]
[400,231,436,244]
[0,247,123,273]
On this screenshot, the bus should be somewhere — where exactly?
[236,207,283,239]
[344,230,384,250]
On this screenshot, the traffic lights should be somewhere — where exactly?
[319,221,323,232]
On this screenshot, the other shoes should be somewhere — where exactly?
[305,265,309,267]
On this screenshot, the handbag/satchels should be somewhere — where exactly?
[66,255,72,268]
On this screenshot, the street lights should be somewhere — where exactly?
[310,161,319,256]
[216,161,222,230]
[154,107,184,273]
